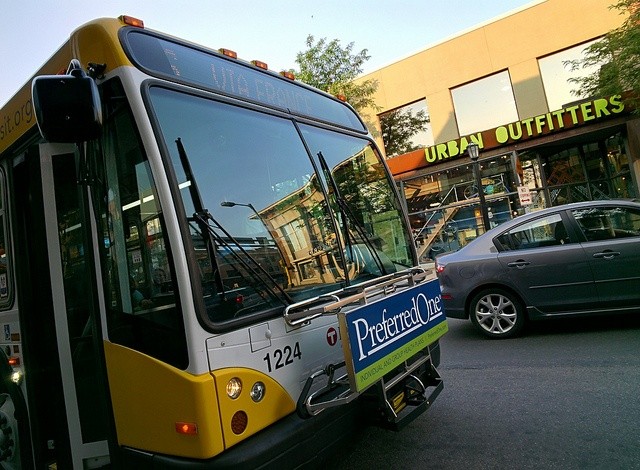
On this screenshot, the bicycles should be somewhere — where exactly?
[431,218,459,252]
[463,173,509,198]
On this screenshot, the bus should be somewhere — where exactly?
[1,15,449,469]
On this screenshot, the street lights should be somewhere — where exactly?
[466,142,491,233]
[220,202,293,291]
[434,199,640,339]
